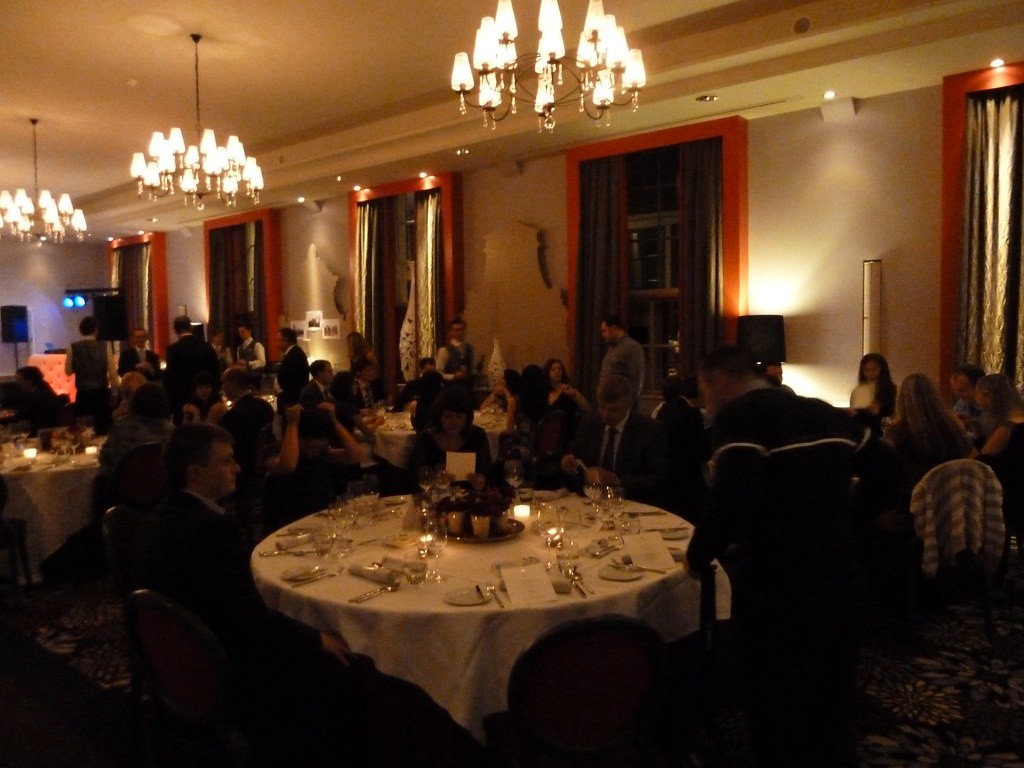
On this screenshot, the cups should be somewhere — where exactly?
[557,542,580,576]
[374,399,504,430]
[403,551,426,586]
[880,417,891,431]
[435,467,451,489]
[56,449,68,463]
[548,561,575,594]
[254,390,275,403]
[518,488,534,503]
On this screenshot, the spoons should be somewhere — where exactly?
[355,583,399,603]
[574,572,594,594]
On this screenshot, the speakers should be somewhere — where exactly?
[1,305,28,342]
[93,295,128,341]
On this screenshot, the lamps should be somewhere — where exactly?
[451,0,646,135]
[64,293,88,308]
[130,34,263,210]
[0,119,87,248]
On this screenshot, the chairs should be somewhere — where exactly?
[509,616,671,768]
[125,590,252,768]
[909,457,1006,639]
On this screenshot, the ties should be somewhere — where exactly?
[323,390,332,402]
[364,387,371,408]
[602,426,619,470]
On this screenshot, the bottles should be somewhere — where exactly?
[457,352,467,371]
[473,354,485,375]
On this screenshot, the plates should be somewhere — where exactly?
[442,587,494,605]
[599,508,690,581]
[424,518,525,543]
[280,496,420,580]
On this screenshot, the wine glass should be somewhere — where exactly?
[6,419,80,462]
[386,395,394,415]
[325,458,625,585]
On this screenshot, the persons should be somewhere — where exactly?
[264,402,363,513]
[403,386,495,492]
[966,374,1024,457]
[273,326,310,415]
[652,376,706,478]
[884,373,974,495]
[16,316,280,526]
[349,360,379,410]
[560,374,669,503]
[300,361,355,466]
[762,358,796,396]
[347,332,377,373]
[687,346,922,768]
[149,423,354,701]
[854,353,897,426]
[600,315,645,414]
[436,318,480,401]
[949,364,986,449]
[395,357,449,433]
[480,358,593,446]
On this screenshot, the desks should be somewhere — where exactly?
[249,489,732,747]
[354,406,507,481]
[0,435,108,560]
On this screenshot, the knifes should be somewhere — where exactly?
[476,584,484,600]
